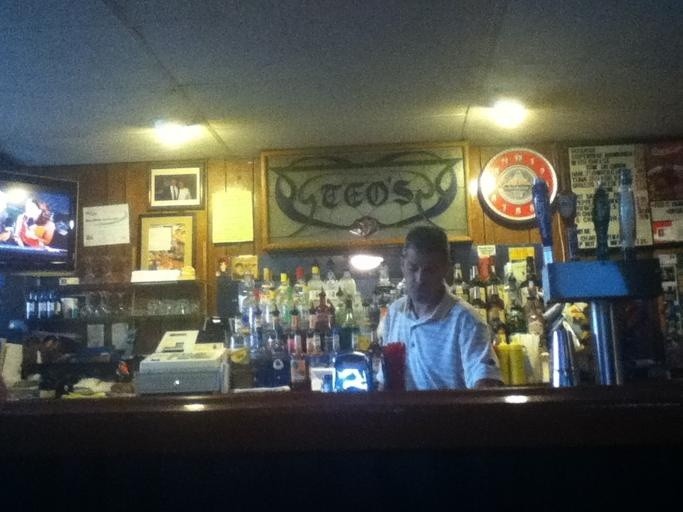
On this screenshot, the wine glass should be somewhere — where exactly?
[80,290,128,321]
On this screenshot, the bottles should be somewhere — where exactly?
[228,259,545,393]
[26,285,61,319]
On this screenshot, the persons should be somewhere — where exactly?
[13,198,55,248]
[176,181,190,200]
[0,192,18,246]
[161,177,179,200]
[215,260,235,317]
[375,226,506,391]
[231,263,254,280]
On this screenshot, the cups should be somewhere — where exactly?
[147,297,191,315]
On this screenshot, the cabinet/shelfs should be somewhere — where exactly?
[24,279,201,366]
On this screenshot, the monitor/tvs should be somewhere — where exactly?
[0,170,80,278]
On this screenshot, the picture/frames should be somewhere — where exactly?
[136,161,205,273]
[259,140,474,253]
